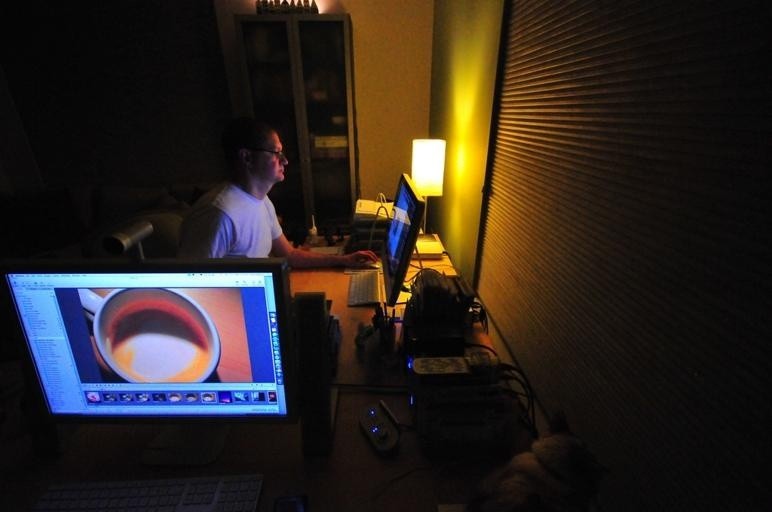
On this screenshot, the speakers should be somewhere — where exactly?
[405,355,518,460]
[403,268,475,351]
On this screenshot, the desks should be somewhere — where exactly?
[1,233,536,511]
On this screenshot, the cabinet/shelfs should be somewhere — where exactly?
[234,14,357,236]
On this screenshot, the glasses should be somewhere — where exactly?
[257,148,283,160]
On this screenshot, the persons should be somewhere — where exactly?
[468,436,602,512]
[164,126,378,266]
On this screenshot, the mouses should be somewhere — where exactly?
[272,486,308,511]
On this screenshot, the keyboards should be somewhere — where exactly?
[30,473,265,512]
[346,269,379,306]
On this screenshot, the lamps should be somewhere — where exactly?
[412,139,445,234]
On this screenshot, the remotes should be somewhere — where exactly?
[412,356,501,376]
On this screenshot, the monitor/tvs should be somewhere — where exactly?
[0,257,292,467]
[378,173,425,307]
